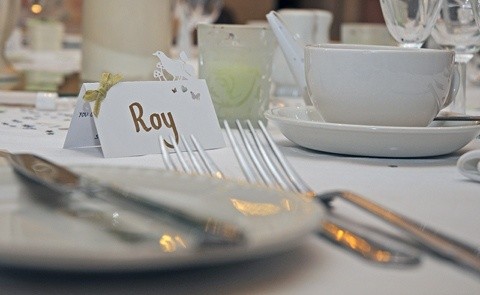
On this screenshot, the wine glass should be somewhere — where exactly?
[430,0,480,116]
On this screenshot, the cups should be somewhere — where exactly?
[270,9,332,95]
[380,0,443,50]
[304,42,461,127]
[196,22,275,129]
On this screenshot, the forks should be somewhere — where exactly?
[223,117,480,275]
[159,134,420,266]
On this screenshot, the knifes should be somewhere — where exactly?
[0,149,246,244]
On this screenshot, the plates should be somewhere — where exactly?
[0,162,327,271]
[264,105,480,158]
